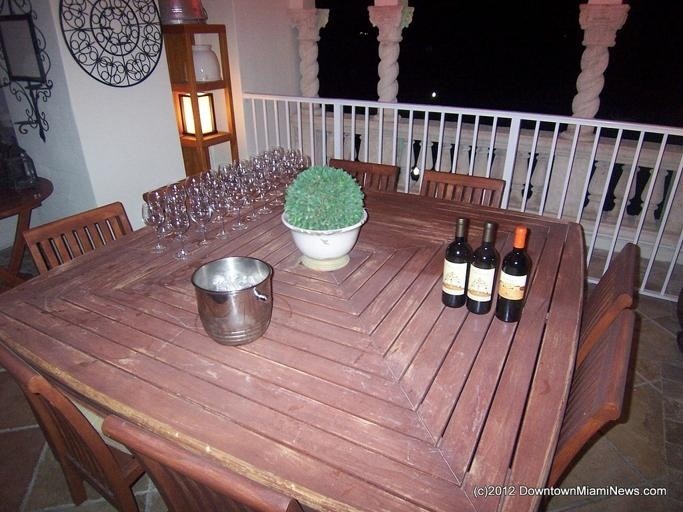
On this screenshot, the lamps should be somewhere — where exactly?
[178,92,218,137]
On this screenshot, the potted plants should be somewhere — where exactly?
[281,165,368,272]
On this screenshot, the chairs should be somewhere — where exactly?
[101,413,304,512]
[420,170,506,209]
[142,170,212,220]
[329,158,401,193]
[575,242,637,369]
[22,202,133,274]
[0,344,146,512]
[544,309,636,487]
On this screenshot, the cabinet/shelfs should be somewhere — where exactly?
[162,24,239,177]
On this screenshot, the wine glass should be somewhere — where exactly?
[141,145,312,261]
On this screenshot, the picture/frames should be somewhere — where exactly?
[0,12,47,84]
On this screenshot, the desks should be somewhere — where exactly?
[0,176,54,294]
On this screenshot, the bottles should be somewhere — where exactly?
[441,216,532,323]
